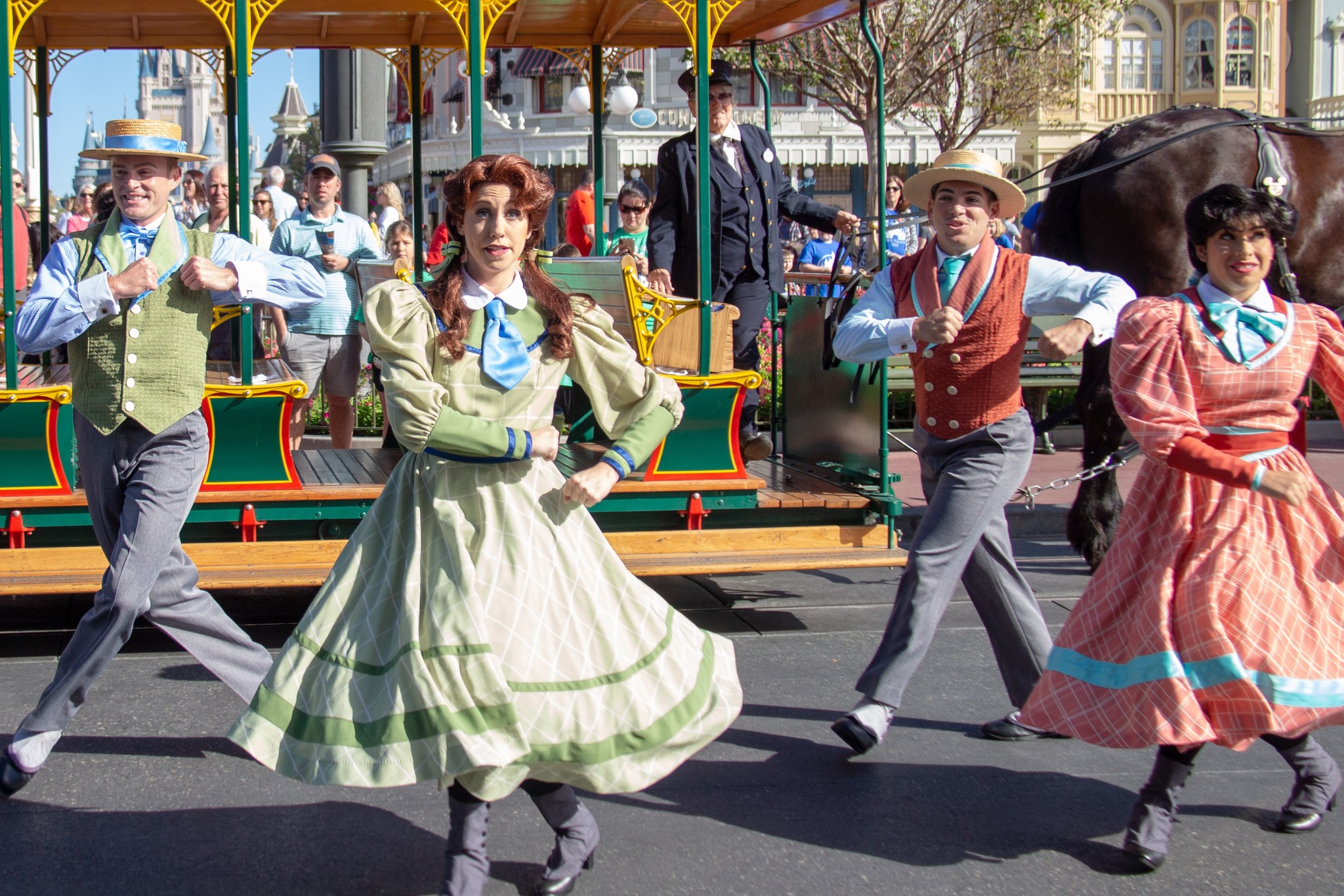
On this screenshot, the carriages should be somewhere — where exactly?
[0,0,1343,624]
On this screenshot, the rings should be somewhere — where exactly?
[653,282,658,285]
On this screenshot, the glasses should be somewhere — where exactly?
[182,180,195,184]
[708,93,732,104]
[80,193,94,198]
[378,192,383,196]
[300,197,309,200]
[13,183,24,188]
[619,202,647,214]
[252,200,270,205]
[886,187,900,191]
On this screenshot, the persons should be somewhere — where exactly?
[370,180,448,283]
[172,169,210,229]
[229,154,743,896]
[589,181,653,285]
[988,201,1043,256]
[269,155,382,414]
[1007,185,1344,869]
[831,149,1136,754]
[647,62,860,467]
[0,169,118,309]
[192,162,309,362]
[565,171,608,257]
[0,119,325,796]
[549,243,582,258]
[777,173,936,301]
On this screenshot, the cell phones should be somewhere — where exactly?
[619,238,635,255]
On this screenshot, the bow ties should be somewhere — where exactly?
[1209,302,1286,363]
[118,222,159,250]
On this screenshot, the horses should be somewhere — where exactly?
[1029,99,1344,579]
[19,216,70,367]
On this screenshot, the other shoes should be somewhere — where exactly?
[980,710,1072,740]
[739,432,773,465]
[0,725,63,796]
[832,699,894,753]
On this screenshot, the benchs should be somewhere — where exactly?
[356,257,412,454]
[0,293,75,497]
[541,255,764,480]
[887,316,1083,456]
[196,304,307,491]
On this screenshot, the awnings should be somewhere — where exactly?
[442,50,500,102]
[399,90,433,124]
[710,29,844,72]
[511,45,643,75]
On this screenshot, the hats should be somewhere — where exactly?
[83,119,208,162]
[304,154,341,178]
[902,149,1026,219]
[678,59,732,98]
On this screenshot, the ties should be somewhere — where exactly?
[718,136,750,174]
[482,298,531,390]
[939,255,971,308]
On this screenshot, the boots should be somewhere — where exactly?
[531,784,600,896]
[1275,737,1342,833]
[1125,751,1194,870]
[444,788,493,895]
